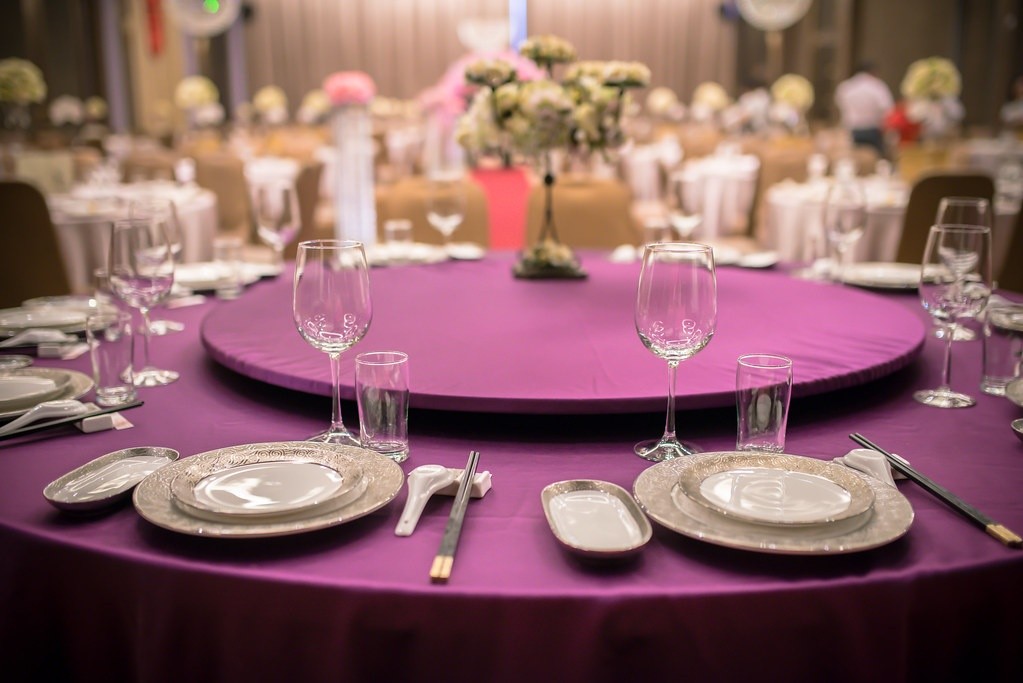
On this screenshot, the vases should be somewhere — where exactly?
[517,152,588,279]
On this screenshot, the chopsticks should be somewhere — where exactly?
[848,433,1023,547]
[429,450,481,583]
[0,401,144,439]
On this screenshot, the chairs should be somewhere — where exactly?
[896,174,1001,266]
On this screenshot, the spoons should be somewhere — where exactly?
[0,400,87,435]
[394,465,454,537]
[0,327,68,346]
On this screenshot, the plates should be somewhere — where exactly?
[632,450,915,556]
[42,441,405,539]
[0,293,120,333]
[540,480,652,560]
[833,263,948,289]
[678,454,876,528]
[136,261,282,292]
[1006,378,1023,406]
[0,354,95,418]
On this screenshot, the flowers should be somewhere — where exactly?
[457,32,646,157]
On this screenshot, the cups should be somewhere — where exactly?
[383,217,414,266]
[981,304,1023,396]
[735,353,793,454]
[355,350,411,464]
[92,263,139,342]
[212,236,249,301]
[87,313,138,406]
[624,148,705,243]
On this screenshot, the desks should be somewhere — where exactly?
[0,246,1023,683]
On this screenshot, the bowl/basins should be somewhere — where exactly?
[1011,418,1023,441]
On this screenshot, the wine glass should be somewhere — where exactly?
[634,240,718,463]
[107,220,179,387]
[127,193,185,337]
[935,195,989,309]
[823,182,868,289]
[292,239,374,447]
[911,225,992,408]
[426,165,465,267]
[248,179,304,279]
